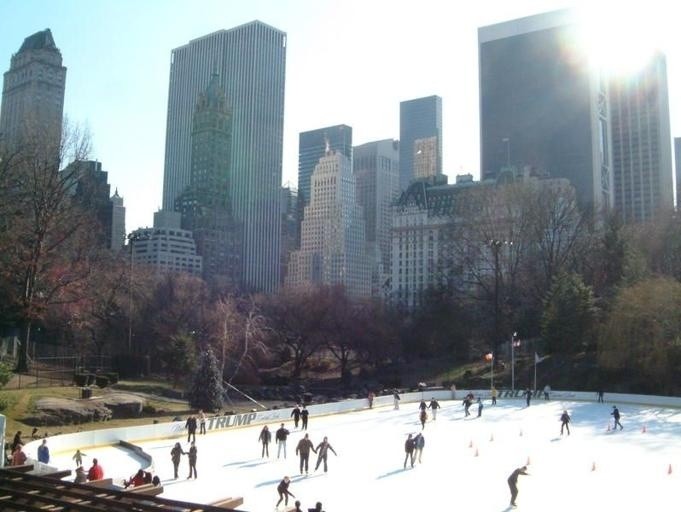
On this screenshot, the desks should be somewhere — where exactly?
[124,232,153,353]
[487,237,514,388]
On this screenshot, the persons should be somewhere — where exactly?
[171,411,206,480]
[596,386,604,403]
[429,397,440,420]
[490,387,497,407]
[523,387,532,407]
[368,389,375,409]
[420,411,427,430]
[393,389,401,410]
[419,399,427,411]
[508,466,528,507]
[611,405,623,431]
[10,430,160,486]
[462,391,483,417]
[560,410,570,435]
[543,384,551,400]
[257,404,337,512]
[413,433,424,464]
[403,434,413,469]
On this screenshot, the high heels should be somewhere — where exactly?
[474,449,479,457]
[469,441,473,448]
[607,424,611,431]
[526,456,530,465]
[642,425,647,433]
[488,435,494,442]
[667,463,673,474]
[519,428,523,438]
[591,462,596,472]
